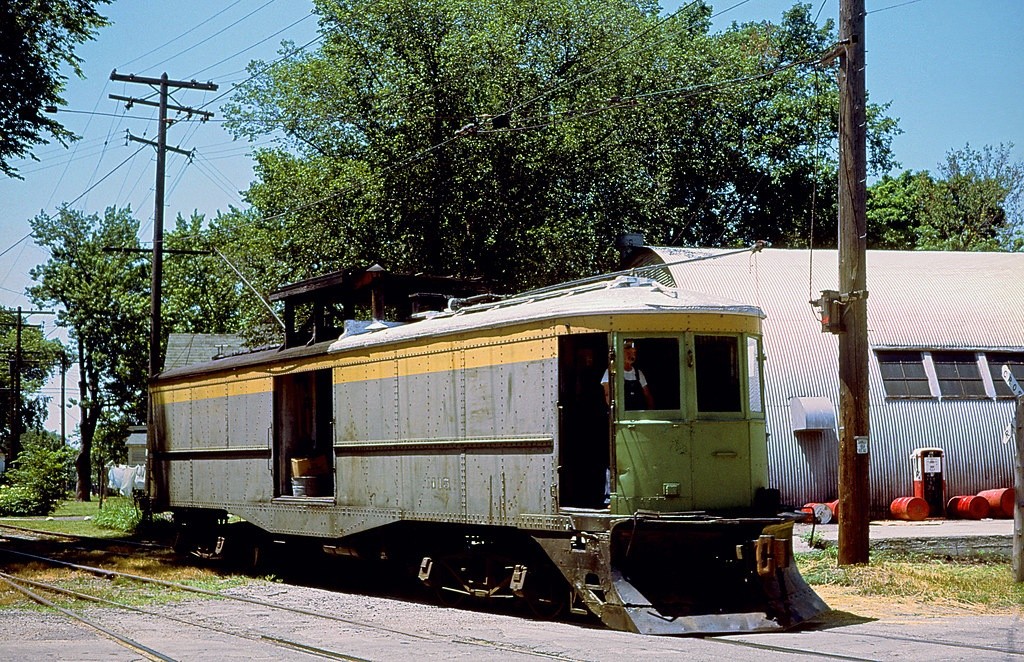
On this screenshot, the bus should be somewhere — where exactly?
[133,242,832,635]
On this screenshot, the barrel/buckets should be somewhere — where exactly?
[947,496,990,520]
[890,496,929,521]
[291,476,317,497]
[802,503,832,524]
[976,488,1015,518]
[823,499,838,521]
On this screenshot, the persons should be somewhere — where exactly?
[600,342,653,508]
